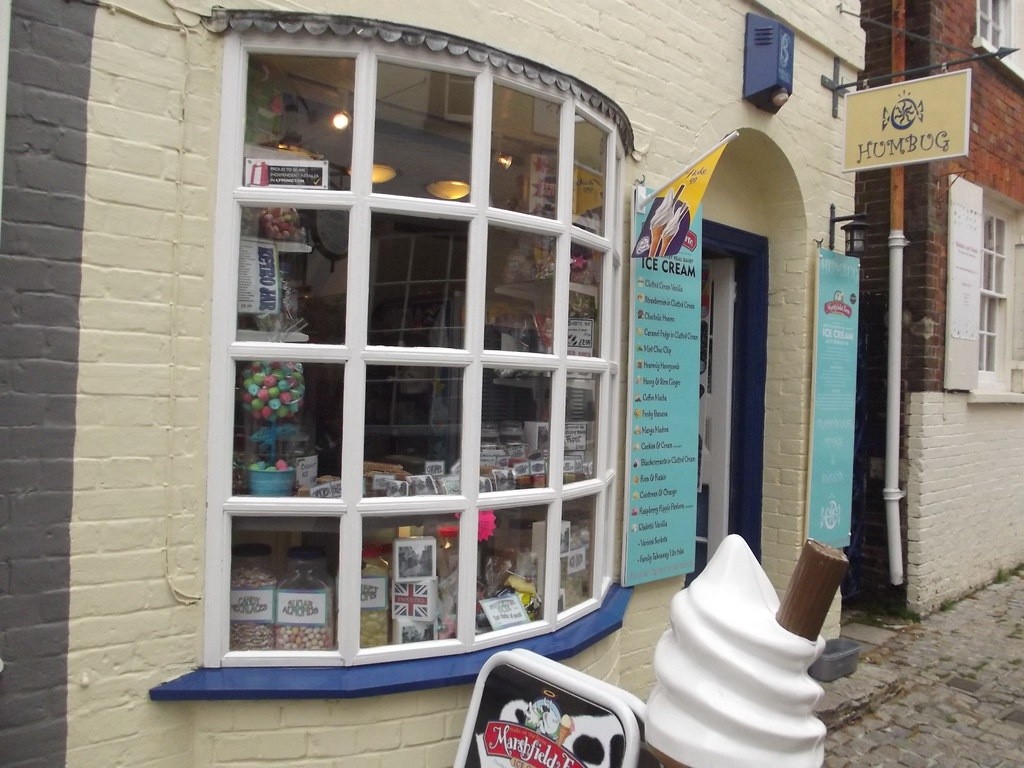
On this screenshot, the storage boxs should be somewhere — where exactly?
[530,520,571,613]
[393,536,437,583]
[524,421,549,450]
[810,638,860,681]
[392,576,440,622]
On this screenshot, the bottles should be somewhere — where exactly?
[436,524,462,636]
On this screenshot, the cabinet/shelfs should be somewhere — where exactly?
[366,230,464,437]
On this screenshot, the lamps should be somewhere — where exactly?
[328,161,404,185]
